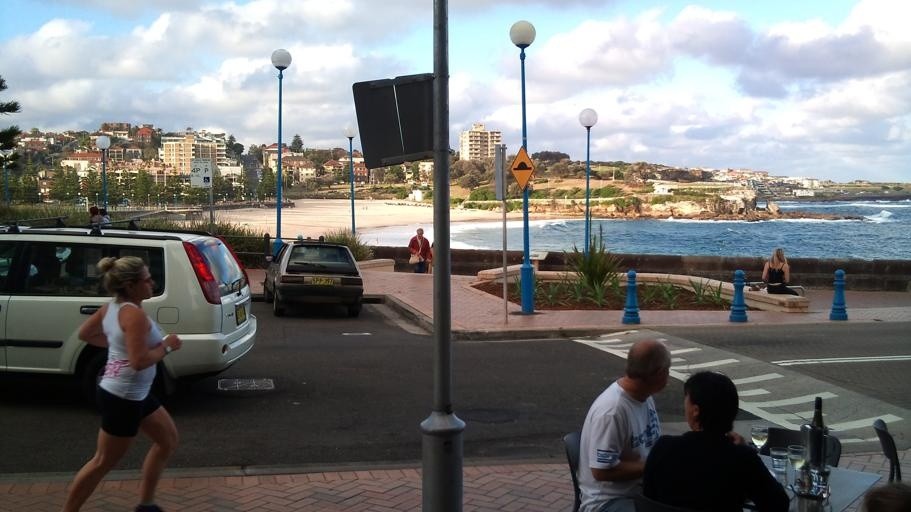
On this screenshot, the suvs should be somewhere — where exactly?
[1,223,258,420]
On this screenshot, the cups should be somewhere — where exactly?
[809,465,831,492]
[766,445,790,476]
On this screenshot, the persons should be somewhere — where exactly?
[635,369,790,512]
[408,228,433,273]
[857,482,911,512]
[762,248,800,297]
[89,206,104,224]
[576,339,672,512]
[61,255,182,512]
[98,208,112,226]
[427,241,433,274]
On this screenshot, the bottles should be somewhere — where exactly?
[788,444,807,491]
[807,397,827,466]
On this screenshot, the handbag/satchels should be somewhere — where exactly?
[408,253,419,264]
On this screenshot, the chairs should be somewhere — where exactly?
[872,417,903,484]
[562,429,605,511]
[754,424,842,468]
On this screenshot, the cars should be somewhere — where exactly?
[264,241,365,320]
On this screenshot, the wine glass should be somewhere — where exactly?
[749,424,768,452]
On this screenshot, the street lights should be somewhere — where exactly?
[344,125,359,251]
[0,139,14,217]
[580,108,598,281]
[96,135,111,216]
[510,19,536,315]
[38,191,274,209]
[271,49,292,262]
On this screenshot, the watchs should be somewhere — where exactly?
[161,342,172,354]
